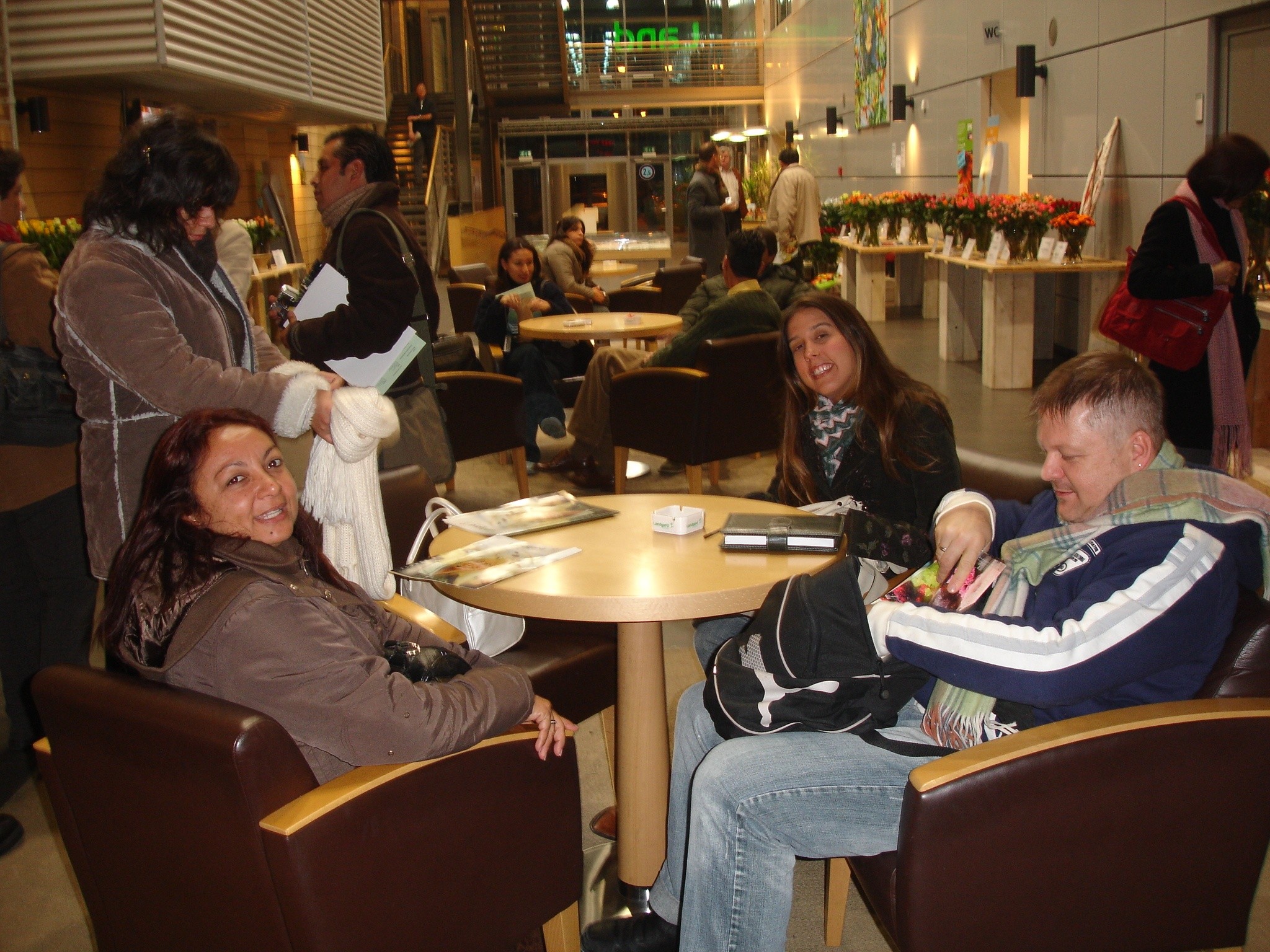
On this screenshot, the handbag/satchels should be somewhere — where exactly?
[704,557,930,738]
[1099,197,1232,372]
[378,387,458,482]
[384,639,473,682]
[399,497,525,658]
[0,338,85,446]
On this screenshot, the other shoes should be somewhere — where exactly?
[537,457,550,470]
[589,806,618,840]
[526,461,537,475]
[579,911,681,952]
[535,448,592,470]
[563,460,613,482]
[540,418,568,438]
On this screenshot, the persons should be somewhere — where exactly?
[471,140,819,488]
[49,112,347,581]
[266,128,456,485]
[589,294,966,841]
[92,401,579,785]
[404,82,437,180]
[1099,133,1270,471]
[0,143,93,856]
[580,348,1270,952]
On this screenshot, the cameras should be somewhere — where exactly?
[268,284,298,321]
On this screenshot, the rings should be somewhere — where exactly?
[550,720,556,723]
[937,543,947,552]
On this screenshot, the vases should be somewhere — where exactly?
[862,221,884,247]
[949,225,971,256]
[1003,230,1025,264]
[252,252,273,272]
[857,221,868,243]
[1023,227,1050,262]
[1061,227,1089,262]
[853,222,861,239]
[904,218,930,245]
[972,223,994,261]
[886,217,902,241]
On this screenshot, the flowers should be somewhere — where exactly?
[241,214,286,254]
[15,216,83,269]
[819,189,1099,233]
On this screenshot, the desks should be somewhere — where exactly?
[522,227,675,275]
[922,250,1127,394]
[516,309,686,483]
[428,489,851,918]
[829,234,938,321]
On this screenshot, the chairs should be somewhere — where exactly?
[823,595,1270,952]
[27,645,589,952]
[604,260,703,311]
[610,325,788,495]
[445,260,497,331]
[617,252,710,288]
[422,345,531,499]
[378,462,616,726]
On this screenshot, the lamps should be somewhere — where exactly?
[784,119,799,143]
[14,96,50,134]
[825,106,843,135]
[891,83,914,123]
[1011,43,1051,100]
[291,132,309,155]
